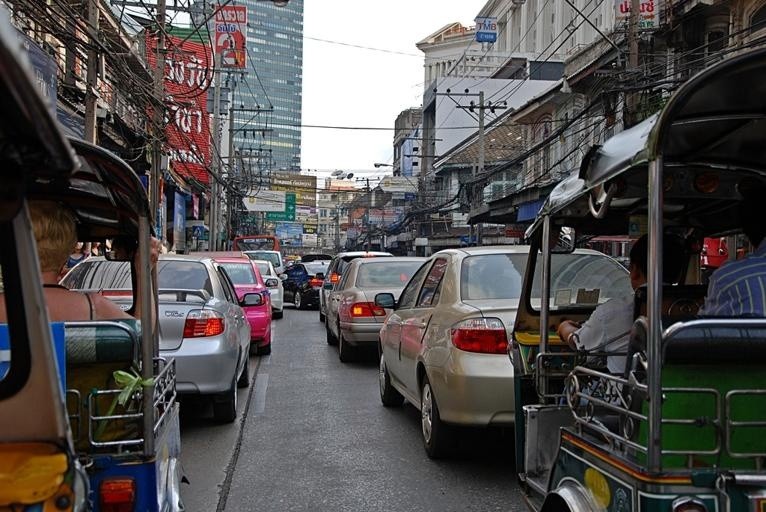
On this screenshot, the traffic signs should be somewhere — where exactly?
[266,194,297,220]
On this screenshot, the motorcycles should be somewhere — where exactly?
[0,7,191,511]
[506,46,766,511]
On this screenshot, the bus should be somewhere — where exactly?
[233,235,280,251]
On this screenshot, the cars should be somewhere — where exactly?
[58,251,289,423]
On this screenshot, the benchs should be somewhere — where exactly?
[62,317,143,452]
[619,313,766,468]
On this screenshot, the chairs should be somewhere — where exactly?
[619,281,708,329]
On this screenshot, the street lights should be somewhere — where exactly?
[374,162,426,206]
[155,0,290,64]
[512,0,639,63]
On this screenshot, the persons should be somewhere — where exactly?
[557,233,691,406]
[0,200,162,334]
[697,200,766,317]
